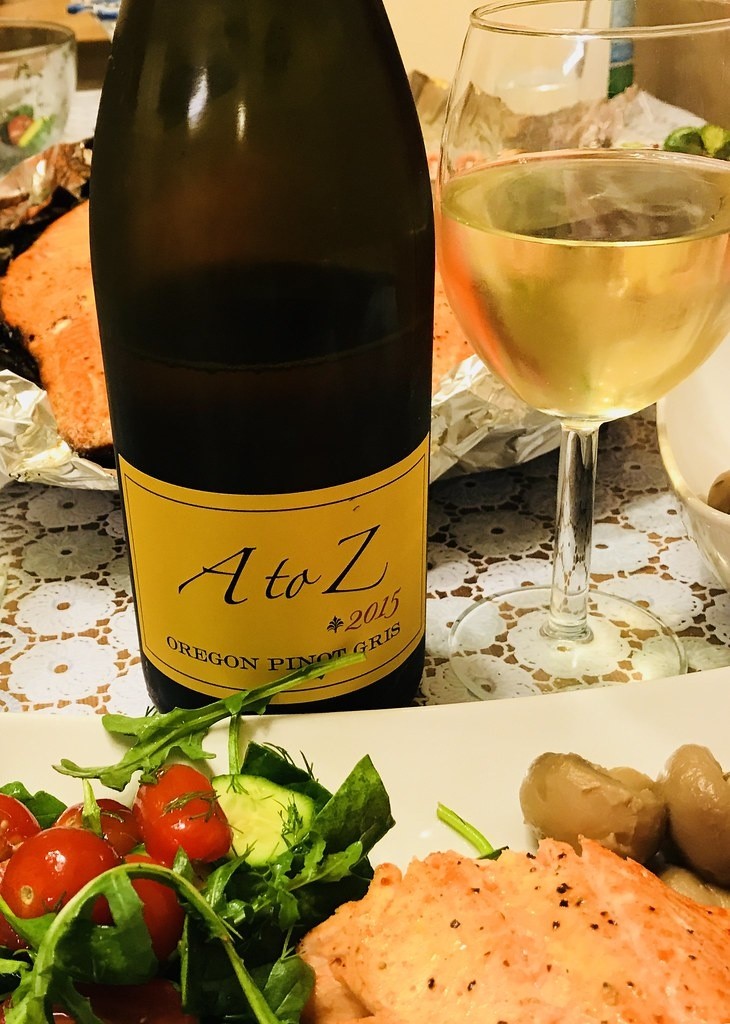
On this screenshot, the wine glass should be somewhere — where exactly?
[435,1,728,702]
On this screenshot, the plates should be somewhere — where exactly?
[3,664,730,881]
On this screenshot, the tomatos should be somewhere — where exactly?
[0,765,236,1024]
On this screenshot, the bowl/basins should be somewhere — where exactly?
[654,331,730,593]
[0,19,77,180]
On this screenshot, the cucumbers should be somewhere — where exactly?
[196,742,408,983]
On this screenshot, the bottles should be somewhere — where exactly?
[86,1,433,715]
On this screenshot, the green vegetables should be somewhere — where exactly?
[52,648,369,792]
[2,795,388,1024]
